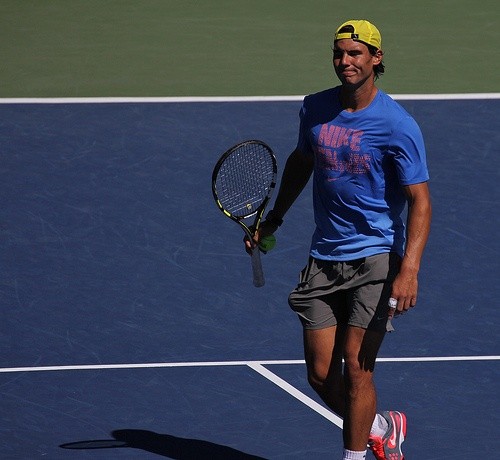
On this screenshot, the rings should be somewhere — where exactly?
[387,297,398,310]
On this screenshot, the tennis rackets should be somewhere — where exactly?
[211,139,279,287]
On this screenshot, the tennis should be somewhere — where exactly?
[259,234,276,252]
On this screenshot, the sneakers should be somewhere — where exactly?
[366,410,406,460]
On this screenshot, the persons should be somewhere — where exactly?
[243,20,432,459]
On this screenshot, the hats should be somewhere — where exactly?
[334,19,381,51]
